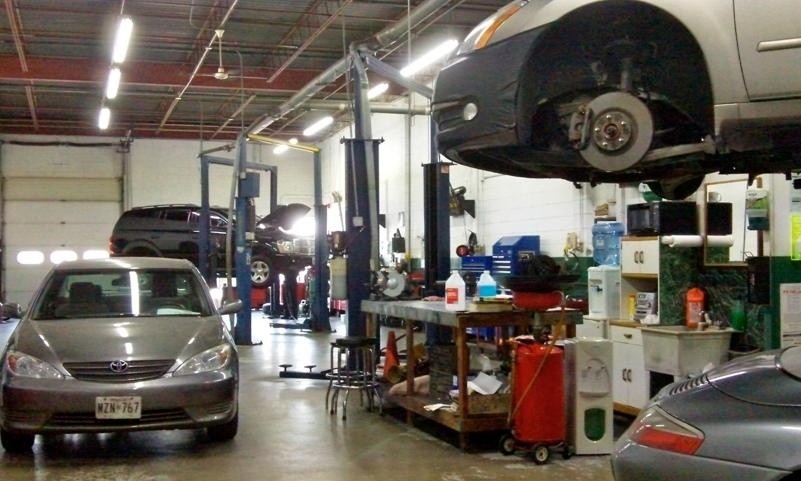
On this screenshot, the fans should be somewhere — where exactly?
[186,30,269,80]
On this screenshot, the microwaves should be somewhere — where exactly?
[625,201,697,237]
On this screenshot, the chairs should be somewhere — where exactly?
[54,282,109,318]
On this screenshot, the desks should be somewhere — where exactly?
[360,296,584,450]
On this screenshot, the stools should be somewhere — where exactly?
[326,335,384,421]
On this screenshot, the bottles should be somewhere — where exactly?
[685,287,706,329]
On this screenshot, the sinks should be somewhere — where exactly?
[641,326,734,375]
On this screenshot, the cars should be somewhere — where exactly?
[430,0,801,204]
[605,343,800,480]
[0,255,248,459]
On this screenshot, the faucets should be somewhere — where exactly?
[699,310,712,324]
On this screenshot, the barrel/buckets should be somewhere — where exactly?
[299,299,306,314]
[591,223,625,265]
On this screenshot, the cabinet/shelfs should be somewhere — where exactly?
[609,236,661,417]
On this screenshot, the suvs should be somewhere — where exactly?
[105,199,316,289]
